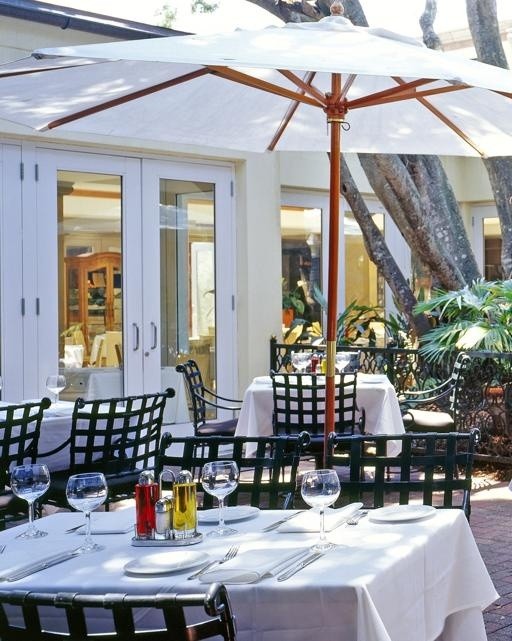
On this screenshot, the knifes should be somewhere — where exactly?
[277,553,323,582]
[262,510,304,533]
[4,553,80,583]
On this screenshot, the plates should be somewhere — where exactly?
[122,551,209,574]
[201,504,260,524]
[371,503,435,522]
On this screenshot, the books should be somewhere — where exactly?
[91,272,106,286]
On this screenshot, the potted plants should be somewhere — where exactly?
[282,277,307,328]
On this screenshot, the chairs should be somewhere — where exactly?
[59,323,215,367]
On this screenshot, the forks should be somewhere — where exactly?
[348,512,369,527]
[186,543,239,581]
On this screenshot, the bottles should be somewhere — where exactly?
[133,471,158,542]
[155,498,173,536]
[172,469,199,533]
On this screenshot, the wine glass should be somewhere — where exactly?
[13,463,49,541]
[63,472,107,555]
[45,374,67,407]
[200,458,240,540]
[301,468,341,551]
[291,351,350,380]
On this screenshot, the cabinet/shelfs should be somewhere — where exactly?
[63,251,124,367]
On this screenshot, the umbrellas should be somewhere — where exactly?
[0,2,512,509]
